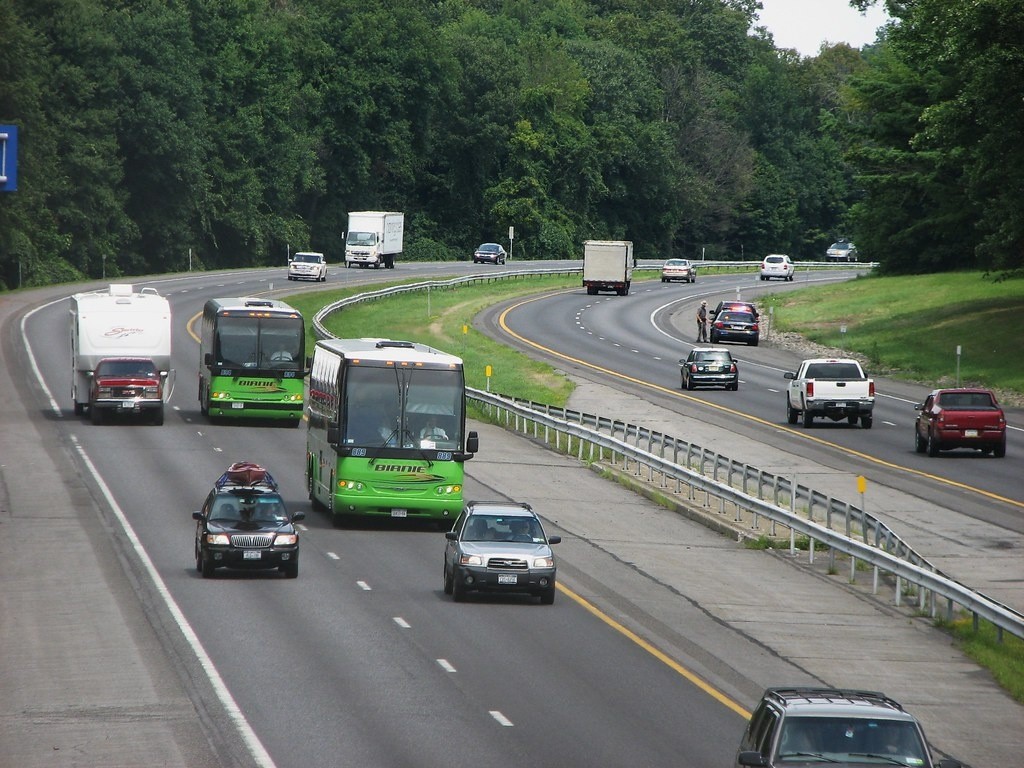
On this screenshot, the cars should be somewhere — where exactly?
[825,240,858,262]
[662,258,696,283]
[760,254,795,282]
[473,243,507,265]
[679,347,739,391]
[710,309,760,346]
[287,251,327,282]
[192,484,306,579]
[709,301,759,320]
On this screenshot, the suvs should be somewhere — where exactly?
[735,686,965,768]
[443,501,562,605]
[914,387,1007,458]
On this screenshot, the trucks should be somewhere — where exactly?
[69,283,176,426]
[341,211,404,270]
[582,239,637,296]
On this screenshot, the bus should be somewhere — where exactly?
[305,337,479,533]
[197,296,313,428]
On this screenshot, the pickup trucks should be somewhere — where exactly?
[783,358,876,430]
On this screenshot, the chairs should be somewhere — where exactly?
[473,519,491,539]
[669,262,686,265]
[218,504,235,517]
[942,396,984,404]
[796,722,881,754]
[808,368,854,378]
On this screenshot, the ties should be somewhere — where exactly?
[431,430,435,441]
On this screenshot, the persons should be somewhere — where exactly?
[882,726,913,756]
[270,341,293,361]
[696,300,713,343]
[506,521,531,541]
[419,415,449,440]
